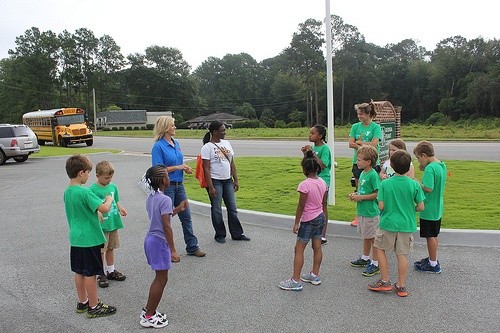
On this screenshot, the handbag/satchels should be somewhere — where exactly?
[195,143,212,188]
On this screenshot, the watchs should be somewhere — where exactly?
[313,155,317,159]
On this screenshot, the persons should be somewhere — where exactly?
[349,103,383,226]
[152,115,206,263]
[278,150,328,291]
[380,139,414,181]
[88,160,126,288]
[406,141,448,274]
[139,164,189,328]
[349,145,383,276]
[301,125,332,246]
[201,121,251,244]
[367,150,426,297]
[63,153,117,319]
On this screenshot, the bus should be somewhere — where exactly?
[22,108,93,147]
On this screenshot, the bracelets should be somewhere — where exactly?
[313,151,315,154]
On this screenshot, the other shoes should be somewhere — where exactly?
[351,217,358,226]
[232,235,250,240]
[215,237,225,243]
[171,259,180,262]
[195,250,206,257]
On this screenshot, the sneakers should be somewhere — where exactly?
[367,279,393,291]
[350,257,371,267]
[85,301,117,319]
[414,260,442,273]
[300,271,322,285]
[106,270,126,281]
[279,276,303,291]
[414,258,429,265]
[76,300,89,314]
[361,264,381,277]
[97,274,109,288]
[139,306,167,320]
[393,283,409,297]
[321,240,328,245]
[140,311,169,328]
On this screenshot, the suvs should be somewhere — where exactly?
[0,123,41,164]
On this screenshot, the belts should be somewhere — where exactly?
[170,181,183,185]
